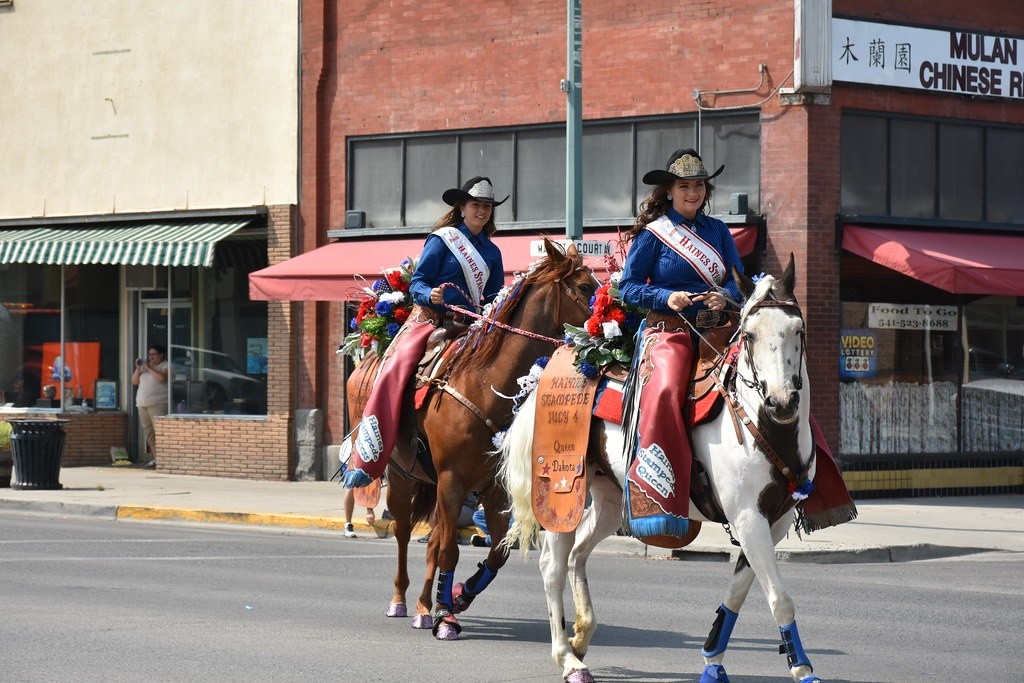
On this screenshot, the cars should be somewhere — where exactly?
[937,347,1018,381]
[166,345,266,409]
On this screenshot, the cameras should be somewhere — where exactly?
[138,360,145,364]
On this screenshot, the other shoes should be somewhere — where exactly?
[146,460,155,467]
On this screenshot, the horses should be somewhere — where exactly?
[346,235,599,641]
[485,252,823,682]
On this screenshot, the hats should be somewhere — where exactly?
[642,148,724,186]
[441,176,510,207]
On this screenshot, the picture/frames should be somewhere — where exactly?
[93,378,119,411]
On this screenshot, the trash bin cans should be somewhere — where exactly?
[6,418,72,491]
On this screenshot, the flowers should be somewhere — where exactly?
[336,253,420,359]
[556,222,656,381]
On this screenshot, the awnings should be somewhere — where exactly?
[1,219,252,268]
[248,242,763,302]
[842,224,1024,295]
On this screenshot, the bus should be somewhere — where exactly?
[0,302,79,408]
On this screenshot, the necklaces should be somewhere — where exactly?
[687,220,698,232]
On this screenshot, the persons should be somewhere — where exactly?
[417,492,478,543]
[619,147,744,516]
[132,345,176,469]
[470,509,514,547]
[354,176,510,459]
[341,462,389,539]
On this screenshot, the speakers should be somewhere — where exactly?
[345,210,365,229]
[730,193,749,214]
